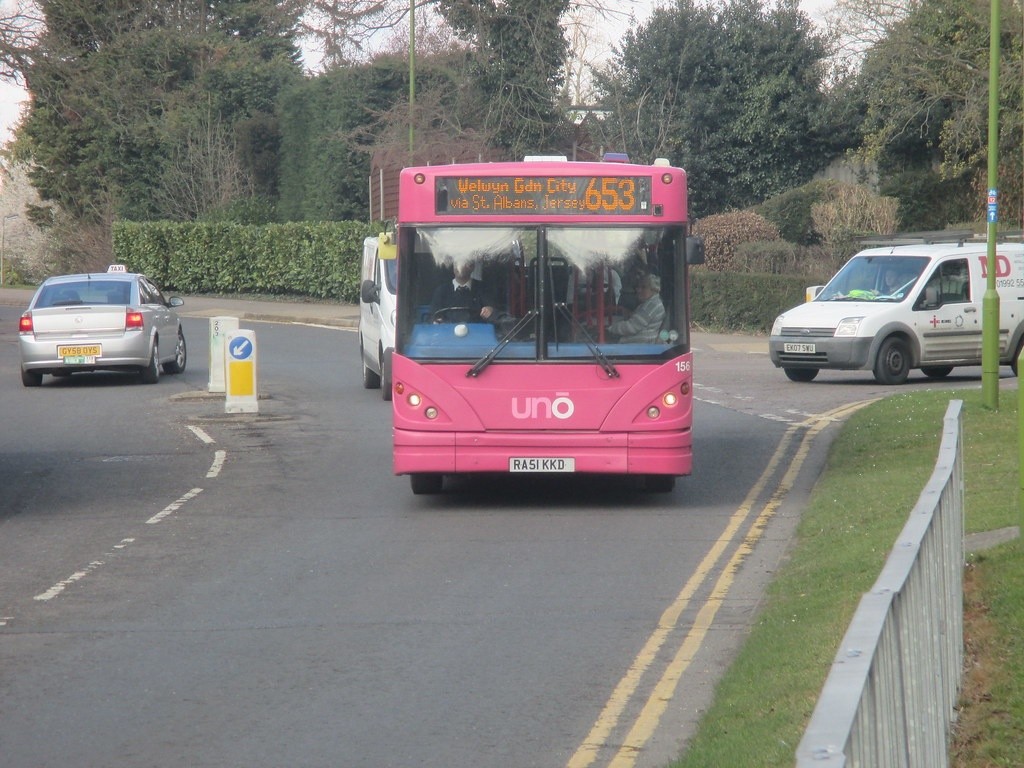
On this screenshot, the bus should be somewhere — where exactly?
[380,149,705,498]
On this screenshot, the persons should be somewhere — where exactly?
[429,252,493,324]
[606,274,666,344]
[872,269,900,297]
[569,250,621,313]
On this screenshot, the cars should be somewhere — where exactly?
[17,264,188,387]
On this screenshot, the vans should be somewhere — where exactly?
[357,219,522,402]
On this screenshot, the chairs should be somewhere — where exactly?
[527,256,568,314]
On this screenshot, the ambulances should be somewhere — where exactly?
[769,234,1024,384]
